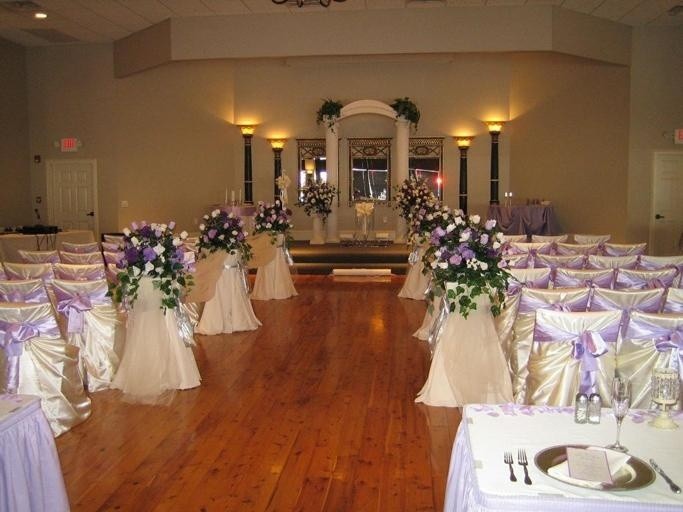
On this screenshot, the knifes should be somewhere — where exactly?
[648,457,681,496]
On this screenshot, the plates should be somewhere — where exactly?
[532,441,658,494]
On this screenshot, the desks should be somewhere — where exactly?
[488,203,557,231]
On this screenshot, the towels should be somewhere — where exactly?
[546,447,632,488]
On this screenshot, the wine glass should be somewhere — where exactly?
[649,367,681,431]
[604,374,632,454]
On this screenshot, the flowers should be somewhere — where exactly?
[387,171,513,322]
[105,176,339,316]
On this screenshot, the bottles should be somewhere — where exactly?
[587,393,601,426]
[573,393,587,424]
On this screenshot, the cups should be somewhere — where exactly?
[544,200,552,206]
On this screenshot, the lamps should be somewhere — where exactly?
[303,157,316,174]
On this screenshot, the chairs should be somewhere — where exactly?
[0,242,120,437]
[493,230,682,406]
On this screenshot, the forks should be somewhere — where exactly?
[517,448,533,485]
[503,450,517,481]
